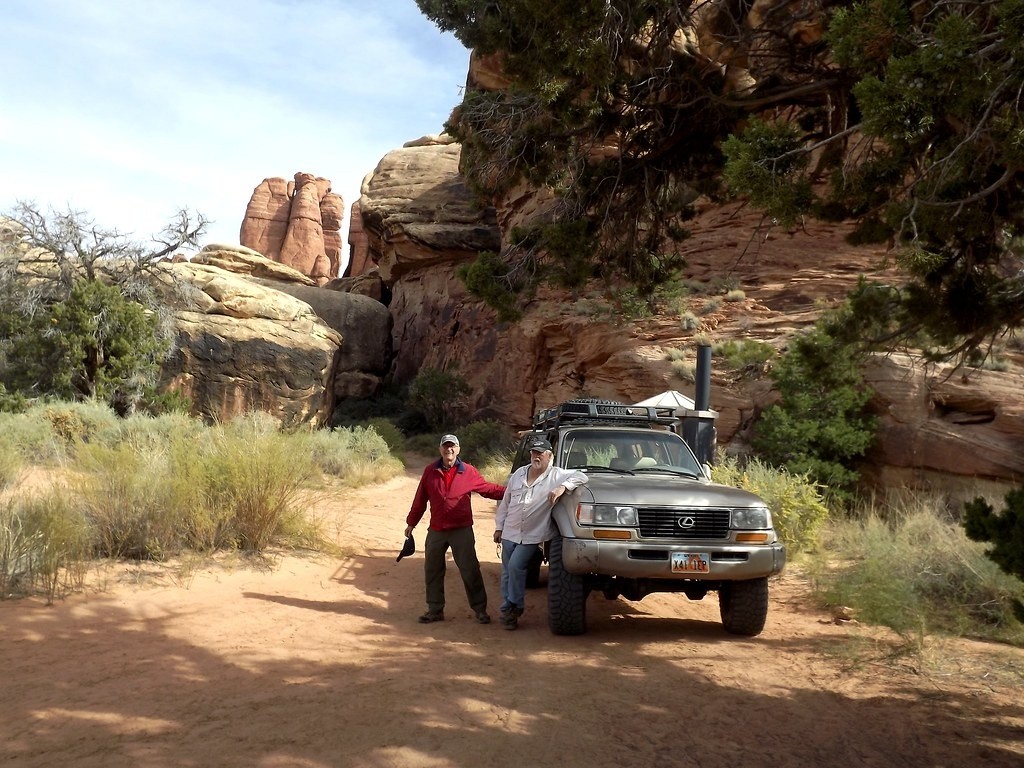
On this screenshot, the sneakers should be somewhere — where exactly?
[503,609,517,630]
[418,610,444,623]
[498,602,524,624]
[475,610,490,624]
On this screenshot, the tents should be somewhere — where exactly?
[632,389,695,435]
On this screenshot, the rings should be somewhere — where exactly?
[552,497,553,498]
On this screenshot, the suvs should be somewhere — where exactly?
[496,400,786,636]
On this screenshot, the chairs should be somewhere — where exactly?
[609,457,657,471]
[563,452,587,467]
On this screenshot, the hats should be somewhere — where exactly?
[396,534,415,562]
[527,439,552,453]
[440,434,459,446]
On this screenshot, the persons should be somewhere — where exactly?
[494,440,589,631]
[405,435,506,623]
